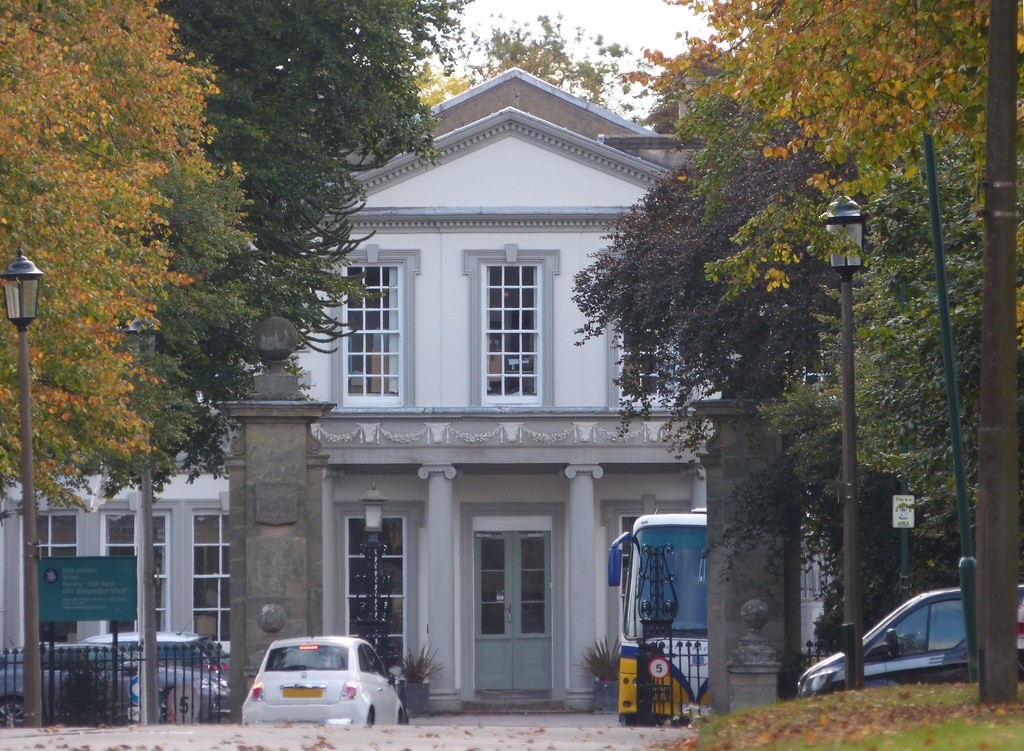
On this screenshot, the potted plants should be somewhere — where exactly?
[583,636,622,714]
[392,644,445,715]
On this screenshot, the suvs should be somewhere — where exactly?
[796,585,1024,698]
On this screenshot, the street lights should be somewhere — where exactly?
[0,245,44,729]
[815,186,868,693]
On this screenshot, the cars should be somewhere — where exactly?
[69,629,230,684]
[0,643,230,728]
[242,635,404,727]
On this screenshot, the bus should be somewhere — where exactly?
[608,506,840,726]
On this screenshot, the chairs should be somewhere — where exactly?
[319,650,345,669]
